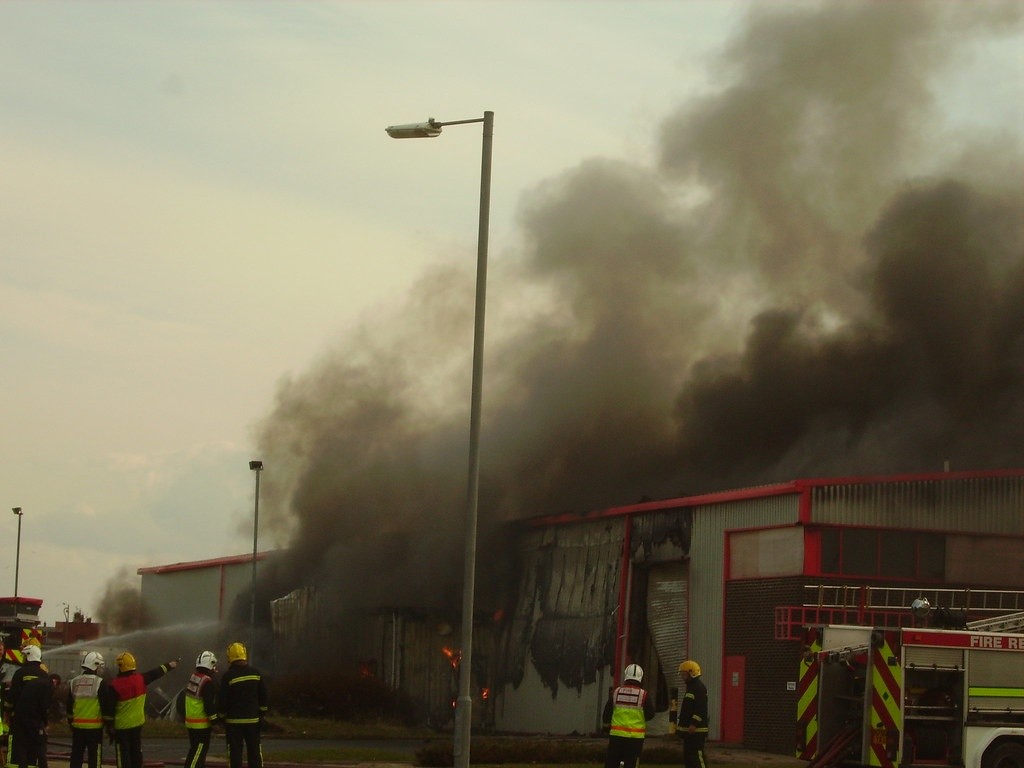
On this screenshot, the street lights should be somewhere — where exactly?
[383,108,498,768]
[247,459,265,667]
[11,506,25,617]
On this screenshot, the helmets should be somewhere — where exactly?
[195,651,217,670]
[624,664,643,683]
[227,642,247,663]
[21,646,42,663]
[116,653,136,673]
[81,651,104,670]
[679,660,701,678]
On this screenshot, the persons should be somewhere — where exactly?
[185,650,223,768]
[602,663,657,768]
[218,643,268,768]
[0,642,178,768]
[676,660,711,768]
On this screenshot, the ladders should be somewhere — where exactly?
[802,585,1024,612]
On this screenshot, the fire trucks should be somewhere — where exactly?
[791,583,1024,768]
[0,595,61,727]
